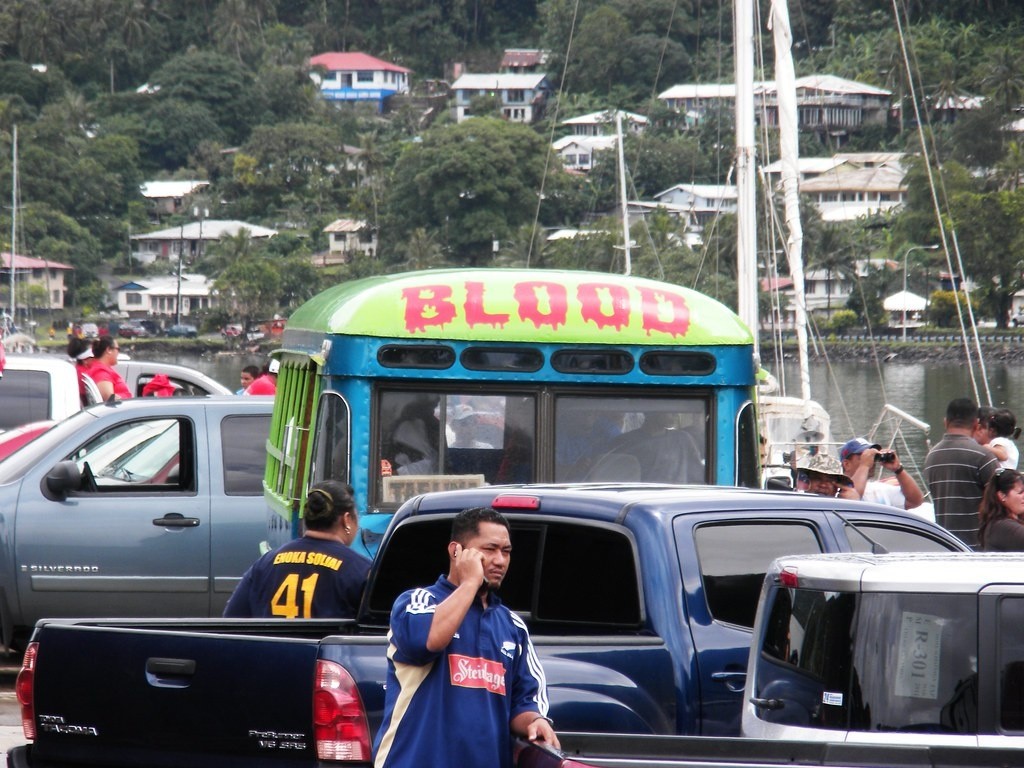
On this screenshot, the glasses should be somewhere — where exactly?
[995,467,1012,490]
[110,346,120,351]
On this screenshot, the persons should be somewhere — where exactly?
[446,405,495,449]
[242,357,281,396]
[921,396,1024,553]
[793,454,861,500]
[68,335,191,407]
[371,506,562,768]
[235,366,260,396]
[838,435,926,511]
[594,408,706,486]
[388,416,438,474]
[758,433,767,472]
[48,326,55,339]
[65,325,109,340]
[977,468,1024,552]
[222,479,374,619]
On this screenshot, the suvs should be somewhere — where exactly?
[118,319,198,337]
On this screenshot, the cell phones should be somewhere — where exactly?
[476,578,490,596]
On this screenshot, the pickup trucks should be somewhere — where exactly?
[8,481,1024,768]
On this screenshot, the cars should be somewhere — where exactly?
[222,324,244,337]
[82,323,99,338]
[2,351,276,630]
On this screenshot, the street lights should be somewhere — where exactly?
[903,244,940,342]
[38,256,54,340]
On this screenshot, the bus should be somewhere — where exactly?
[264,267,760,559]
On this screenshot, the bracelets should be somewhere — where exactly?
[895,465,905,474]
[533,716,554,727]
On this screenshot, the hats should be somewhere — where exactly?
[797,452,854,488]
[839,438,882,463]
[448,405,479,425]
